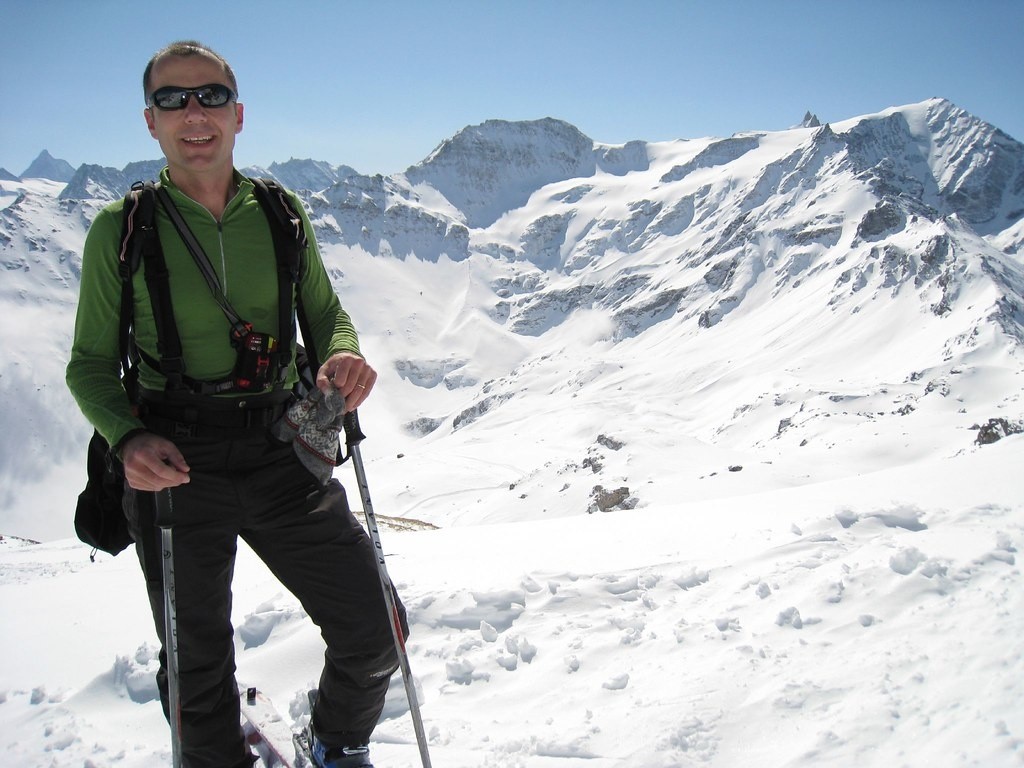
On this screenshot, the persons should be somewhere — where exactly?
[64,40,410,768]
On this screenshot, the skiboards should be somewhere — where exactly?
[239,657,321,768]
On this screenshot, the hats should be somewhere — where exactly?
[270,379,344,486]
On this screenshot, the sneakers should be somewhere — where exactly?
[293,689,373,768]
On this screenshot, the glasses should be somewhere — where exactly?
[149,83,236,111]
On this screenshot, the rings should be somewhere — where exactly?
[356,383,366,390]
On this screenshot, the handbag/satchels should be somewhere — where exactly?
[73,358,135,563]
